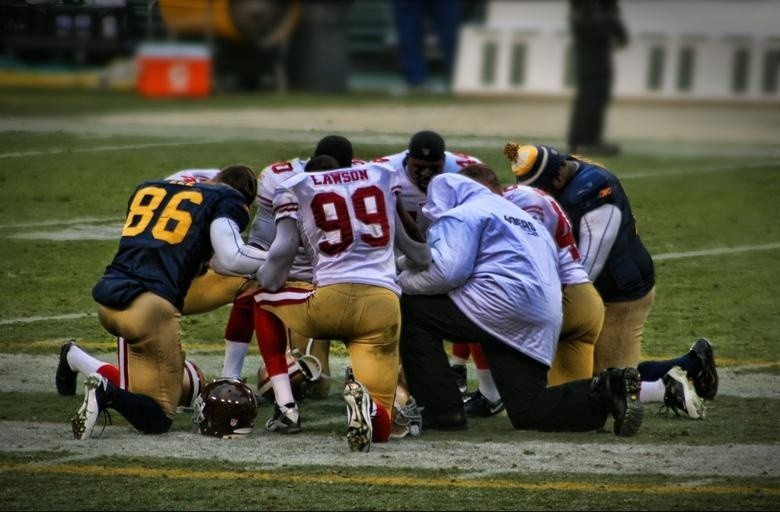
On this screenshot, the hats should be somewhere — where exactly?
[503,140,563,189]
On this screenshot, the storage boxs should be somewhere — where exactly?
[134,40,211,99]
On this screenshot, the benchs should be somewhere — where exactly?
[158,1,265,70]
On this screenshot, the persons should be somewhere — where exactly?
[571,1,630,157]
[55,133,720,450]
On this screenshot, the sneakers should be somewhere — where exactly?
[465,390,509,422]
[265,401,301,434]
[451,363,468,392]
[340,380,375,453]
[55,338,79,396]
[599,338,718,437]
[70,371,114,439]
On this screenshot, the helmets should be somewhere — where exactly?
[179,360,207,410]
[390,385,424,439]
[194,378,257,439]
[257,346,324,403]
